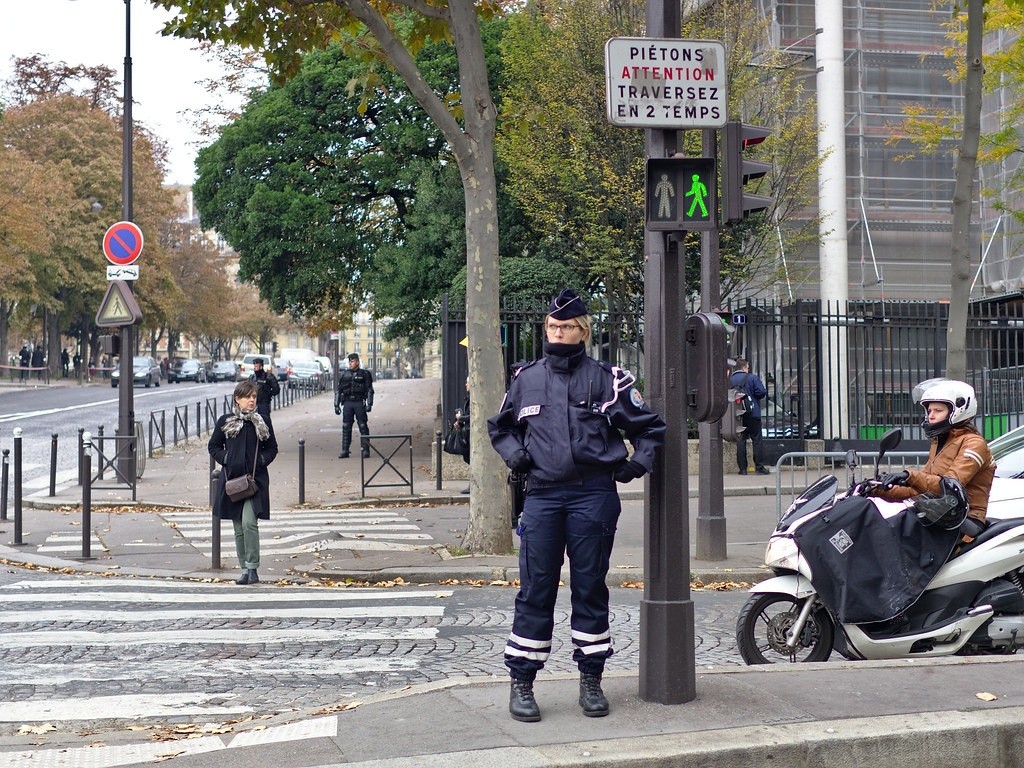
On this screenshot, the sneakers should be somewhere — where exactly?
[739,467,747,475]
[755,464,768,475]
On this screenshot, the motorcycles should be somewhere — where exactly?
[733,423,1024,666]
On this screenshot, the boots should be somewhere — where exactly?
[509,677,541,721]
[580,671,608,717]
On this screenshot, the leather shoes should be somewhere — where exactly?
[236,574,248,584]
[247,569,258,584]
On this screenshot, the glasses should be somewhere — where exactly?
[545,323,581,334]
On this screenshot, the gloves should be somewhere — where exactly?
[609,463,636,484]
[510,450,531,475]
[335,406,342,415]
[366,405,371,412]
[877,472,910,491]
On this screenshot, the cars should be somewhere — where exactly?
[111,356,162,388]
[760,398,818,439]
[206,347,333,391]
[167,359,207,383]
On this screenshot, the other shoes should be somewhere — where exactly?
[461,486,469,494]
[338,450,350,459]
[362,451,370,458]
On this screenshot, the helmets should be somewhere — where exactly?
[903,476,970,531]
[920,379,977,437]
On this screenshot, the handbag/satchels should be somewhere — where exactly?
[444,421,468,457]
[225,473,259,503]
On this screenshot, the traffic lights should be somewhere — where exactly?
[721,118,776,225]
[645,156,718,231]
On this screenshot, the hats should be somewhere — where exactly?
[253,359,263,364]
[548,288,588,320]
[348,353,358,360]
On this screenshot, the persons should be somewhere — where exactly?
[32,345,44,380]
[402,359,413,379]
[208,381,279,585]
[61,348,70,378]
[730,358,771,475]
[334,353,375,457]
[455,375,471,493]
[73,352,81,380]
[237,364,242,372]
[102,356,109,381]
[484,290,664,723]
[247,358,280,418]
[18,345,30,384]
[159,357,169,381]
[88,356,97,382]
[859,377,998,637]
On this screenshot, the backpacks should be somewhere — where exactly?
[731,373,755,415]
